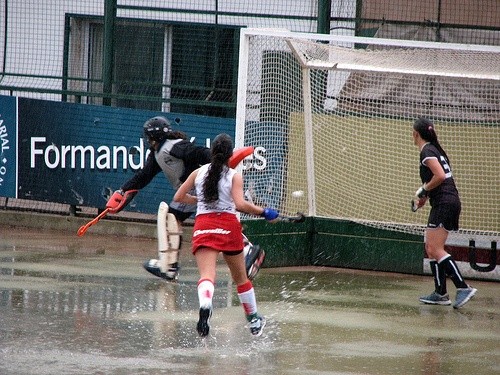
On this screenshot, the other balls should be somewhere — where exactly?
[293,190,305,198]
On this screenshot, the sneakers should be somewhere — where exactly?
[419,291,452,305]
[453,284,478,308]
[145,259,180,280]
[197,304,213,337]
[243,245,266,280]
[249,315,266,337]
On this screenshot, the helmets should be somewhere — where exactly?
[144,116,172,141]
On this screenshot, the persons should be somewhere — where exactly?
[105,116,265,281]
[411,117,478,310]
[173,133,280,338]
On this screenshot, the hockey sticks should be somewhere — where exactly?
[77,208,109,237]
[235,207,306,221]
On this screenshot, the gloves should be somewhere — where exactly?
[260,207,280,220]
[416,187,429,200]
